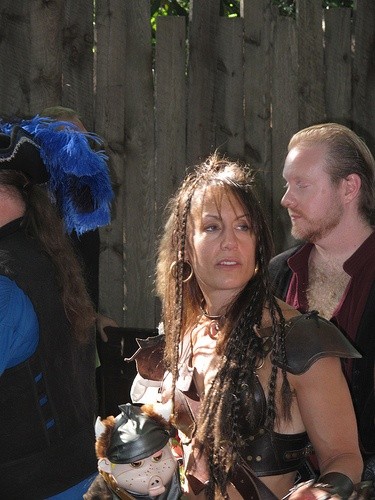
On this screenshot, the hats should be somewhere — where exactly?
[0,118,113,240]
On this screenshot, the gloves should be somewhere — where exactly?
[94,312,119,342]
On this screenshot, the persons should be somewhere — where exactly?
[265,124,375,500]
[150,156,363,500]
[0,106,120,500]
[83,402,177,500]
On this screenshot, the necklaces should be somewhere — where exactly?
[200,298,228,340]
